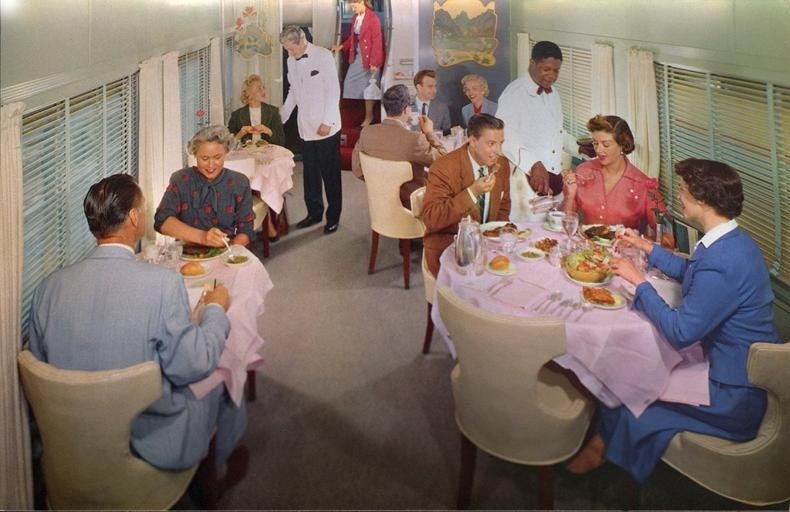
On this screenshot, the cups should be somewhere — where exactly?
[548,210,566,229]
[408,111,421,127]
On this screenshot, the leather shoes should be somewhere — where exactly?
[214,445,248,496]
[567,433,607,475]
[297,215,321,228]
[324,224,338,233]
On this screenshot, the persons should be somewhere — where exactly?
[421,112,512,280]
[349,83,449,256]
[403,68,452,136]
[459,72,499,127]
[562,156,781,510]
[561,114,671,242]
[152,125,257,251]
[495,41,596,215]
[329,0,385,130]
[226,74,285,243]
[277,24,344,235]
[27,173,252,487]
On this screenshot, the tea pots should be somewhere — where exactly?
[529,189,559,215]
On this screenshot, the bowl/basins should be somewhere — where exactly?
[565,261,611,280]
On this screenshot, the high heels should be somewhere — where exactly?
[268,233,280,242]
[355,118,377,130]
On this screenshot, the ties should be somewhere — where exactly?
[478,168,485,226]
[422,103,427,115]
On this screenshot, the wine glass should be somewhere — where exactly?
[561,211,580,245]
[499,227,517,258]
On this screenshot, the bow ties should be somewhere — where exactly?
[537,87,552,96]
[296,54,308,60]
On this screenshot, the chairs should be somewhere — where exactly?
[664,339,788,505]
[410,185,437,354]
[359,152,426,290]
[437,285,596,509]
[251,196,272,259]
[17,350,207,507]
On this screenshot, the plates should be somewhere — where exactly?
[528,236,560,255]
[178,262,212,280]
[478,220,519,242]
[222,255,250,267]
[516,247,546,262]
[578,224,620,248]
[485,262,517,275]
[563,269,615,286]
[176,243,233,261]
[542,222,566,234]
[580,286,628,310]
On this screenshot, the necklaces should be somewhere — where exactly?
[603,162,625,189]
